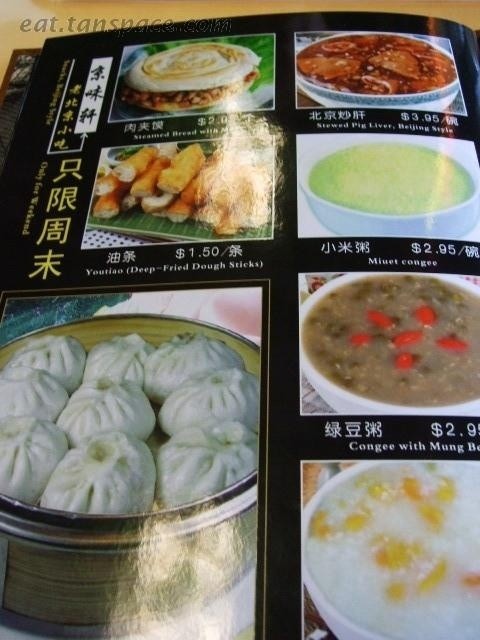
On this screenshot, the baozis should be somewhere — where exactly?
[0,332,260,515]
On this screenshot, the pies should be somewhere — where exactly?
[117,43,261,111]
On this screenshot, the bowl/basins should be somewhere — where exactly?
[297,33,459,109]
[299,134,480,237]
[302,458,479,638]
[299,274,480,417]
